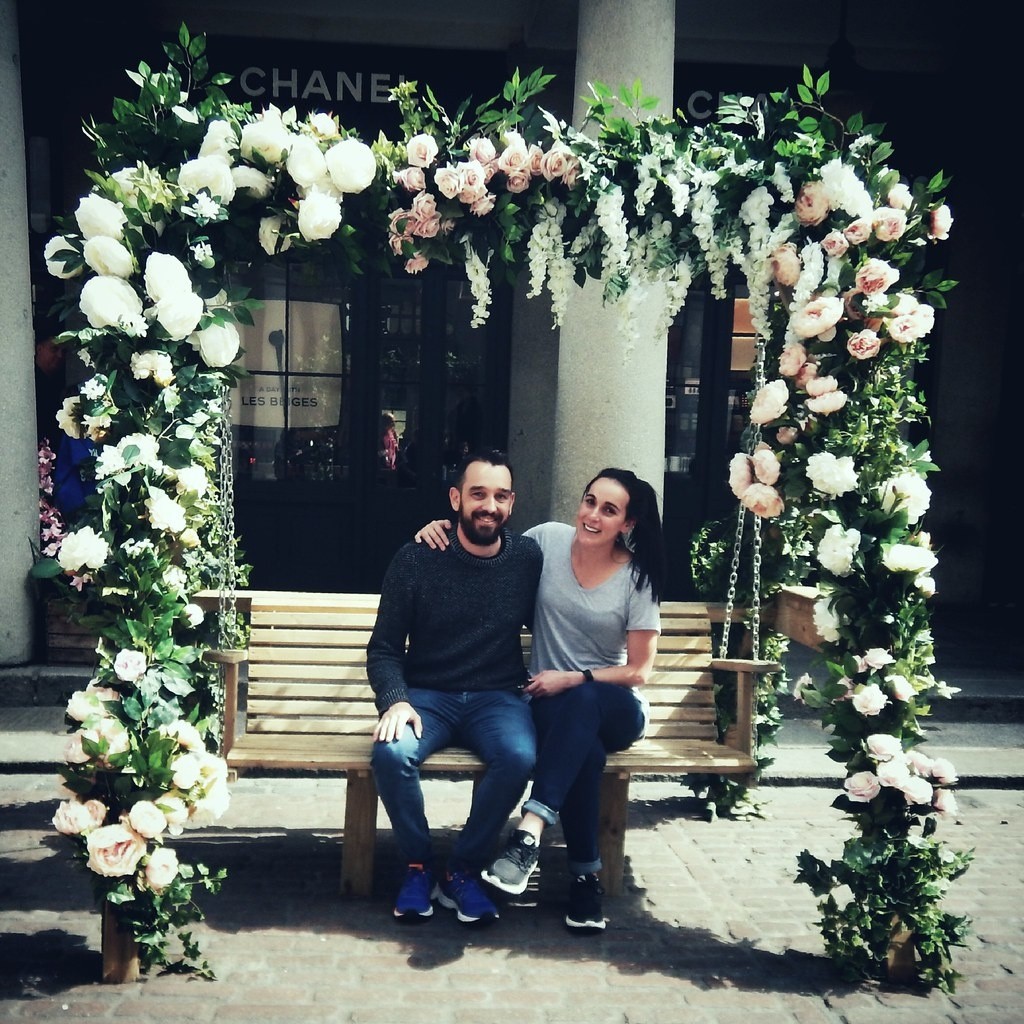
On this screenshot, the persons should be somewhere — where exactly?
[378,412,402,469]
[275,428,314,480]
[411,467,667,930]
[33,321,100,589]
[364,448,546,924]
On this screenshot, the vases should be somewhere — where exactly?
[44,597,114,668]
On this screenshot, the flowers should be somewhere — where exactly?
[28,20,976,997]
[36,434,69,544]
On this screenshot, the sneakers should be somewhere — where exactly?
[480,827,541,895]
[438,872,500,924]
[565,874,606,930]
[393,863,439,919]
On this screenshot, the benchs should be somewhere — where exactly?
[192,588,781,892]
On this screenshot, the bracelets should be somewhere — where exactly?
[582,669,594,684]
